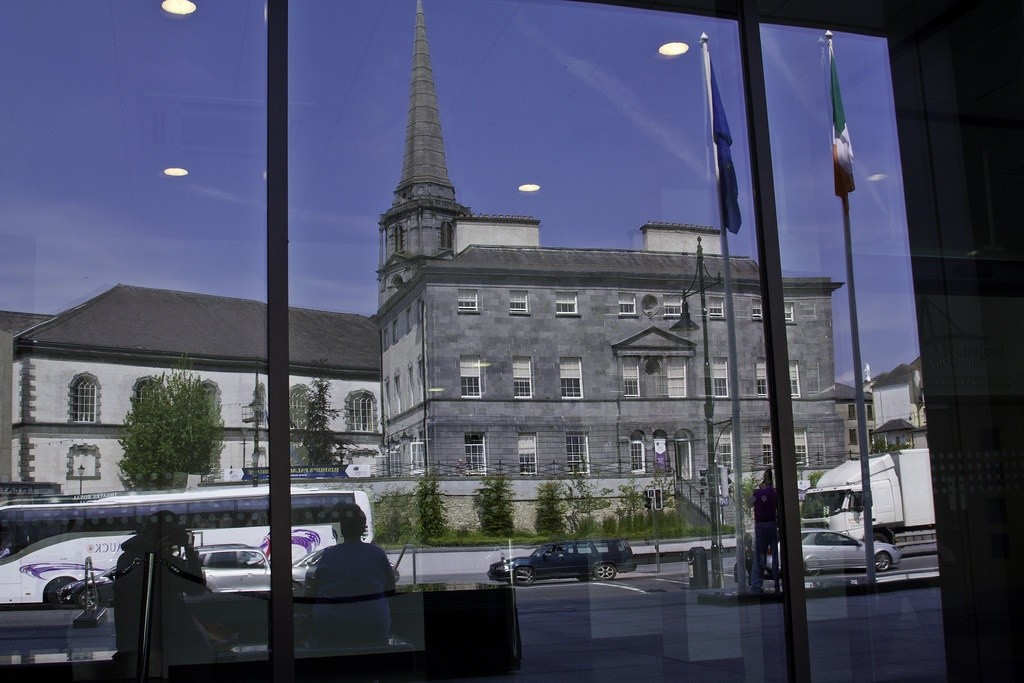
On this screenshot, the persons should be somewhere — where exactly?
[113,511,237,667]
[311,507,395,650]
[747,469,782,596]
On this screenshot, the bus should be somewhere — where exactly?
[0,484,373,606]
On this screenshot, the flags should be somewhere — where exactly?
[830,49,856,195]
[705,52,742,235]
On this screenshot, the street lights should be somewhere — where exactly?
[248,396,267,489]
[670,273,724,589]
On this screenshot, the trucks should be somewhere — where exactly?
[799,449,937,545]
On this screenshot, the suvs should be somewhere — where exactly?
[488,537,638,586]
[182,544,273,596]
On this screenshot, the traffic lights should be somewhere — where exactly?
[643,491,653,511]
[654,488,663,511]
[701,469,710,500]
[720,467,732,498]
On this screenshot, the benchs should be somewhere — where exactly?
[215,638,414,663]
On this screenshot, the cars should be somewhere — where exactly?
[55,564,117,606]
[293,546,400,595]
[765,528,902,577]
